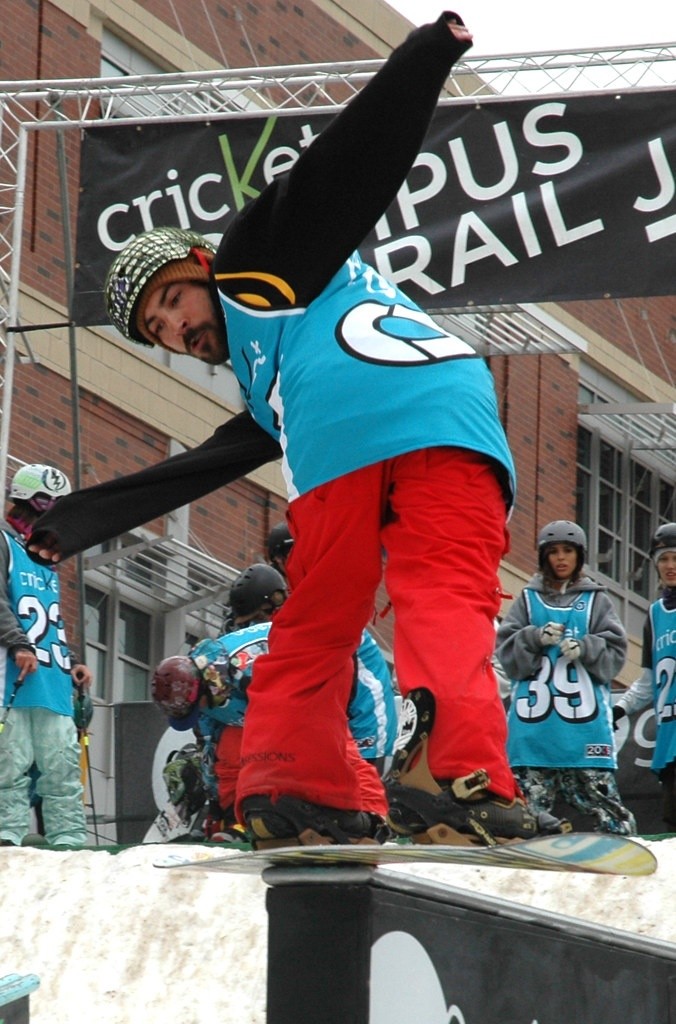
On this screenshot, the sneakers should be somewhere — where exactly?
[394,781,560,837]
[241,794,412,840]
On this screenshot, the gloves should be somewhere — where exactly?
[540,622,566,646]
[613,705,625,732]
[560,637,581,661]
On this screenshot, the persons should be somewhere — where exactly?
[0,463,95,845]
[493,518,638,836]
[149,518,398,845]
[20,9,544,852]
[611,520,676,832]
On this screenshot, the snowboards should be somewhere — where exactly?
[151,830,659,879]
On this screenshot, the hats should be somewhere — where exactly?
[169,704,200,731]
[135,247,215,354]
[234,591,284,624]
[652,547,676,565]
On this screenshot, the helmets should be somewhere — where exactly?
[8,464,72,500]
[151,656,200,719]
[105,227,217,348]
[230,563,287,624]
[537,520,587,571]
[649,522,676,559]
[268,522,294,559]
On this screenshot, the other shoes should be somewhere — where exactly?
[206,815,246,842]
